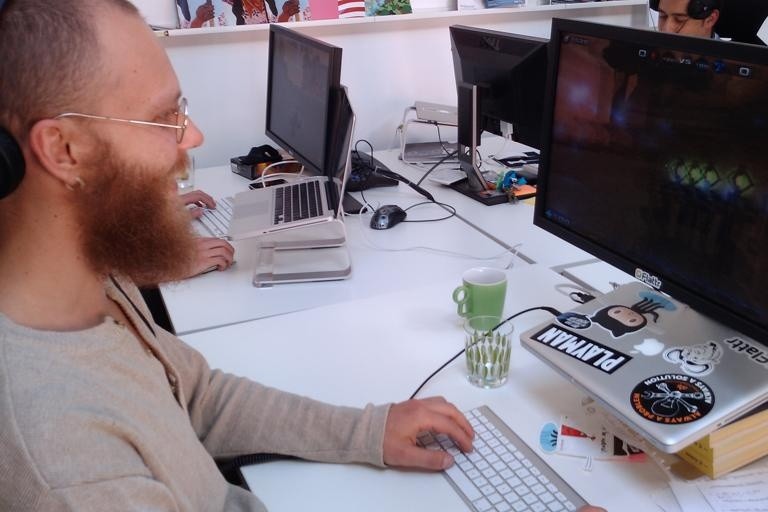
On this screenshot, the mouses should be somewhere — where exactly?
[370,205,407,231]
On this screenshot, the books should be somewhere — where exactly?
[677,402,768,481]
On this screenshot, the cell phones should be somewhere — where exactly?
[248,178,287,190]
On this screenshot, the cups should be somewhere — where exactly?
[180,156,196,191]
[465,316,514,388]
[454,268,509,330]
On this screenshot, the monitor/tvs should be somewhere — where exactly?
[263,24,369,217]
[448,23,551,206]
[531,16,768,344]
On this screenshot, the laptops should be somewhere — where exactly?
[521,281,767,453]
[228,88,355,241]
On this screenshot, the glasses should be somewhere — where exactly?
[52,98,189,144]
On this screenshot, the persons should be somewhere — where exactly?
[648,0,720,39]
[132,189,235,286]
[0,0,610,512]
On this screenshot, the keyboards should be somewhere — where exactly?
[192,196,229,239]
[418,404,588,512]
